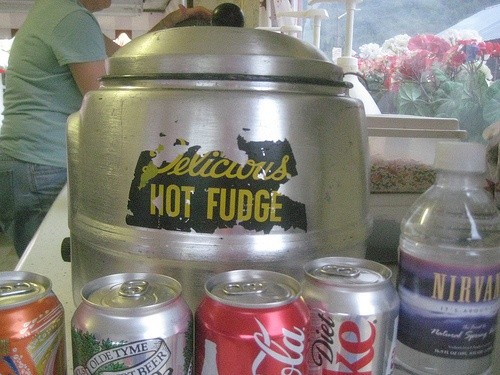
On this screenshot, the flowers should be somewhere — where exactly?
[351,28,500,144]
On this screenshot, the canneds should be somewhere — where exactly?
[295,256,400,375]
[72,272,196,375]
[194,269,312,375]
[0,270,69,375]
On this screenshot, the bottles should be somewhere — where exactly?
[390,140,500,375]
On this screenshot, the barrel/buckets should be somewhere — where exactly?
[67,3,372,309]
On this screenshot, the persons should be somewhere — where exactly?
[0,0,213,260]
